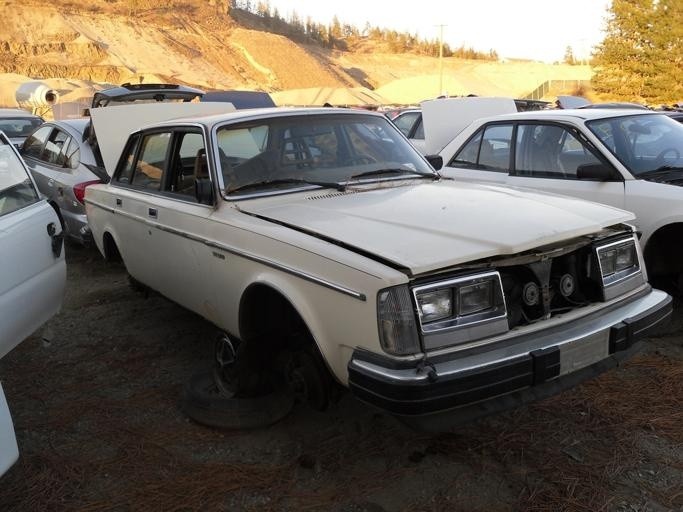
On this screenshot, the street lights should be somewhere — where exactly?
[435,25,449,94]
